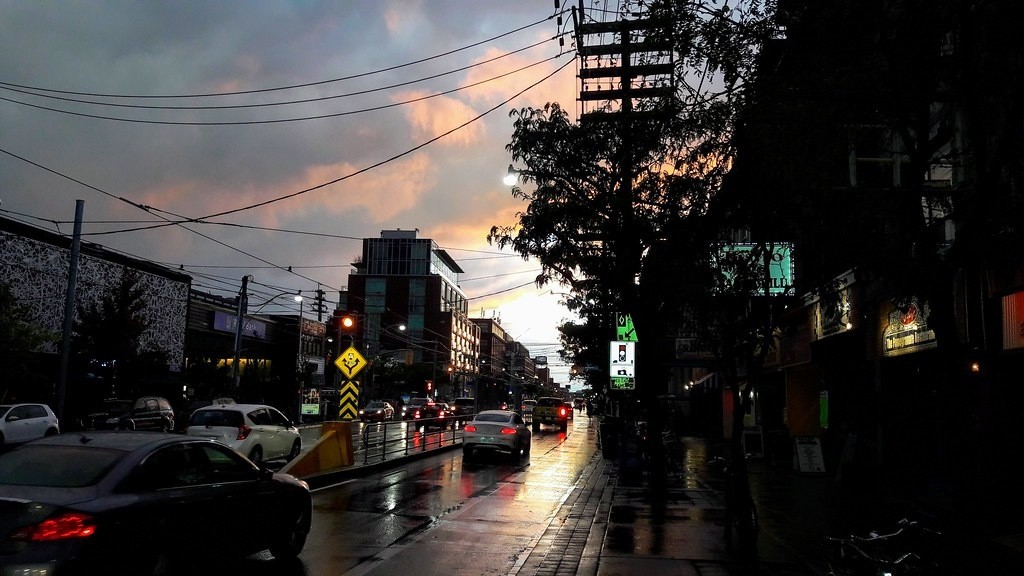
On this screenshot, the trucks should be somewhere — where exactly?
[574,397,584,410]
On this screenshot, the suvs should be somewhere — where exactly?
[80,395,177,432]
[402,396,433,422]
[531,396,567,433]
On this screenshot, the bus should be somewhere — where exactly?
[453,397,475,415]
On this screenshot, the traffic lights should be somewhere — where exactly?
[427,382,432,391]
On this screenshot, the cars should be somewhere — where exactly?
[461,410,532,466]
[520,400,537,414]
[358,402,395,422]
[187,403,302,466]
[0,431,314,576]
[0,403,61,449]
[413,402,455,431]
[564,402,573,421]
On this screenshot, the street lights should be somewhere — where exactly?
[229,275,303,402]
[370,323,406,402]
[335,316,353,390]
